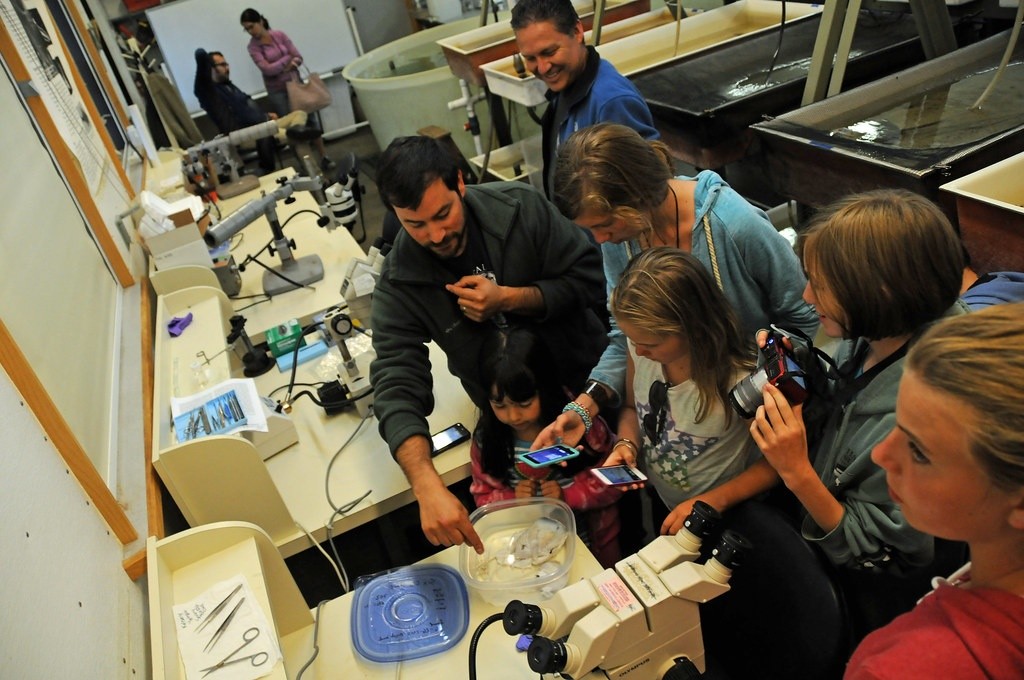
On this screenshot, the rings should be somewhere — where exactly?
[463,307,466,311]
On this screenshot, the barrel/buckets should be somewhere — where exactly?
[340,10,548,182]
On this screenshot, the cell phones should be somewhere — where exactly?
[518,442,579,468]
[430,423,471,458]
[590,465,648,486]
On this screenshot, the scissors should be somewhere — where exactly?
[197,344,236,366]
[183,412,200,436]
[200,627,268,679]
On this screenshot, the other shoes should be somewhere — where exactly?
[322,156,336,168]
[286,125,324,140]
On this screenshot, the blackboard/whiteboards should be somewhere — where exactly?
[145,0,365,119]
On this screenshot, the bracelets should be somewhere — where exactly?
[578,382,608,414]
[613,438,639,457]
[562,401,593,434]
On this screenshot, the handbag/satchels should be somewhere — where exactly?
[285,57,333,115]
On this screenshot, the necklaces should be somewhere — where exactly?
[643,183,680,250]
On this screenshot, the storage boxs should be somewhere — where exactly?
[435,0,1023,272]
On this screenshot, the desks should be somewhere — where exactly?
[149,518,604,680]
[152,285,480,555]
[137,148,376,343]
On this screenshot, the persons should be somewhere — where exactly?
[651,106,923,210]
[528,123,820,467]
[510,0,661,219]
[751,187,964,635]
[917,194,1024,316]
[370,136,609,555]
[601,247,806,536]
[194,8,336,176]
[469,326,620,570]
[842,302,1024,680]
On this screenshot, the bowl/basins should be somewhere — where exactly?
[459,496,577,606]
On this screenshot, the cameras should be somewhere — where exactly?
[726,336,811,419]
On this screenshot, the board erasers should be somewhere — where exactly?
[331,65,344,74]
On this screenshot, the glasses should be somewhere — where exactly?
[243,23,256,31]
[640,380,673,446]
[212,61,229,68]
